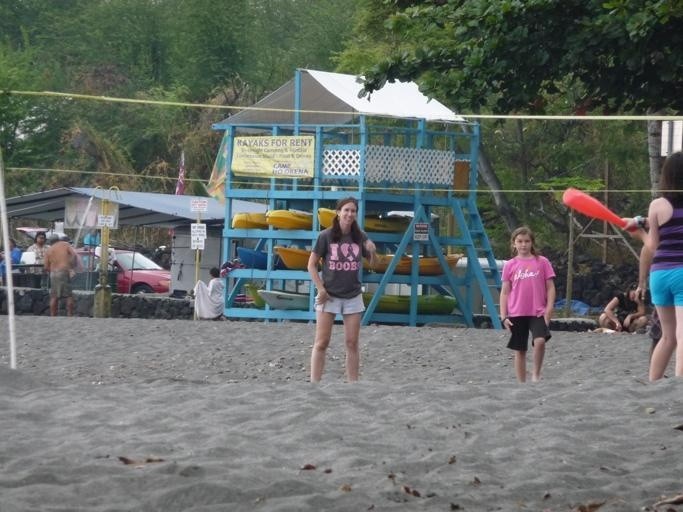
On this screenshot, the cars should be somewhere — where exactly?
[14,223,173,296]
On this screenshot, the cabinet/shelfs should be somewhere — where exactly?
[223,188,475,329]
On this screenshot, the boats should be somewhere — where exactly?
[229,207,461,319]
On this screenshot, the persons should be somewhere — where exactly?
[498,227,557,383]
[598,282,648,335]
[620,216,671,380]
[0,238,21,279]
[193,267,224,319]
[306,197,378,383]
[619,151,682,381]
[26,230,46,264]
[43,233,83,317]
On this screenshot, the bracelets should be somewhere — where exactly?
[500,318,507,323]
[632,216,646,228]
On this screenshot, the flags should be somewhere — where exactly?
[165,158,184,236]
